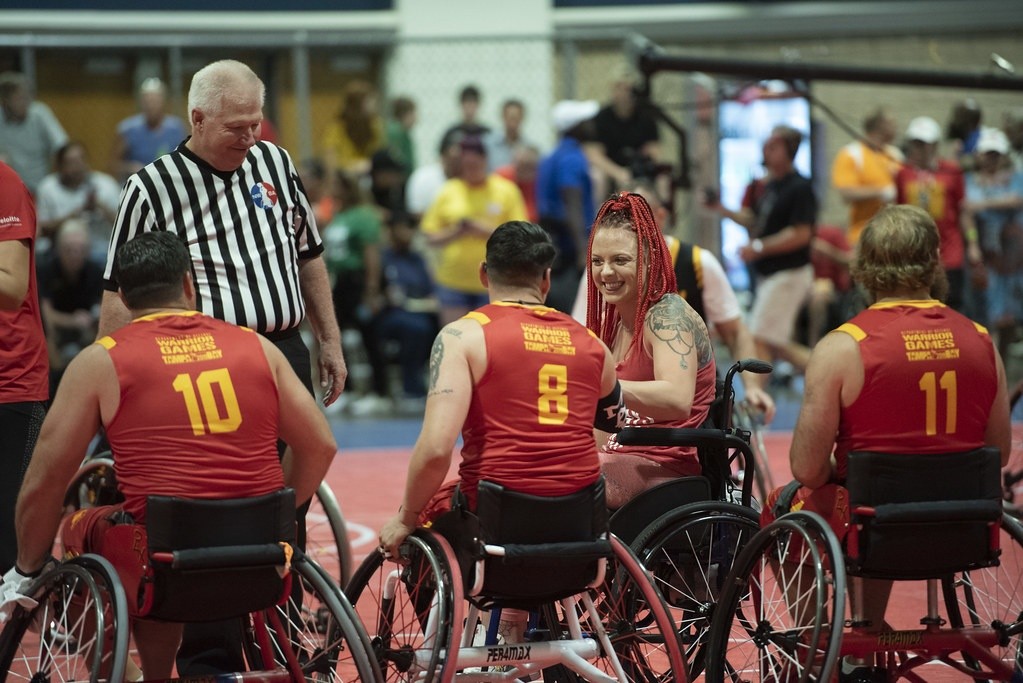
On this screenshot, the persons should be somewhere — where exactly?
[0,161,52,624]
[378,219,627,682]
[699,125,814,388]
[630,186,776,425]
[92,57,345,683]
[805,98,1023,413]
[298,65,677,420]
[759,204,1013,683]
[583,192,717,508]
[1,229,338,683]
[112,75,190,184]
[1,79,123,414]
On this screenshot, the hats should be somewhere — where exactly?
[977,128,1011,156]
[550,99,600,132]
[904,115,942,144]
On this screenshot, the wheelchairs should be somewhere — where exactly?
[705,473,1023,683]
[0,448,390,683]
[318,497,699,683]
[575,357,779,683]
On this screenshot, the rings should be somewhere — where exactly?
[379,546,385,554]
[384,550,393,558]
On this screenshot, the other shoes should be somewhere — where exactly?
[838,656,875,683]
[351,393,394,418]
[400,395,427,414]
